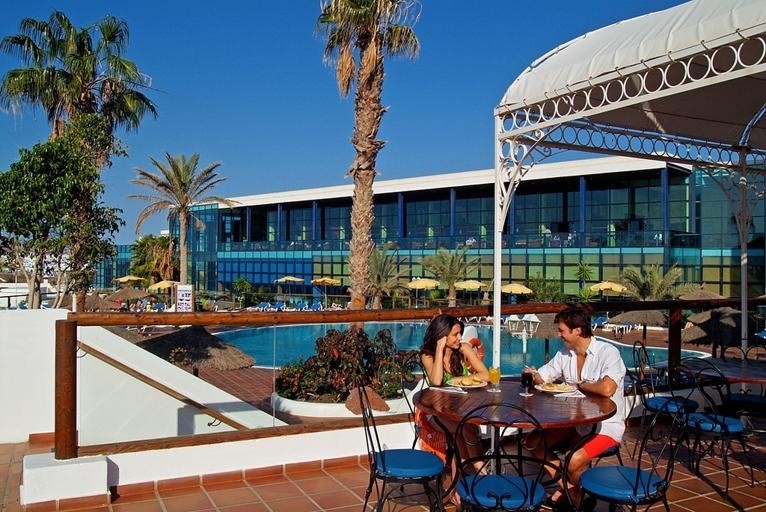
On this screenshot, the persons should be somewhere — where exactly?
[111,296,166,313]
[521,304,629,510]
[418,314,490,507]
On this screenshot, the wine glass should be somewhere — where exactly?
[487,367,502,392]
[519,372,535,396]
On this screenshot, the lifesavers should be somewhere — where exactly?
[463,338,484,373]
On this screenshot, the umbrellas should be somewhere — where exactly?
[589,281,627,302]
[453,279,487,304]
[407,278,441,306]
[311,276,342,294]
[501,283,533,294]
[112,274,146,283]
[150,280,180,290]
[273,275,304,293]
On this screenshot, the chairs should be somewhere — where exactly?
[665,358,756,501]
[710,342,765,446]
[574,394,687,511]
[453,402,563,511]
[255,301,322,312]
[127,302,167,336]
[631,342,700,460]
[400,353,464,489]
[352,376,445,511]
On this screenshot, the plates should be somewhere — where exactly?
[534,382,577,394]
[446,376,488,389]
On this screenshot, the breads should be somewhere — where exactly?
[460,376,483,386]
[544,383,554,390]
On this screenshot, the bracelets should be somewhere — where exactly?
[579,380,587,385]
